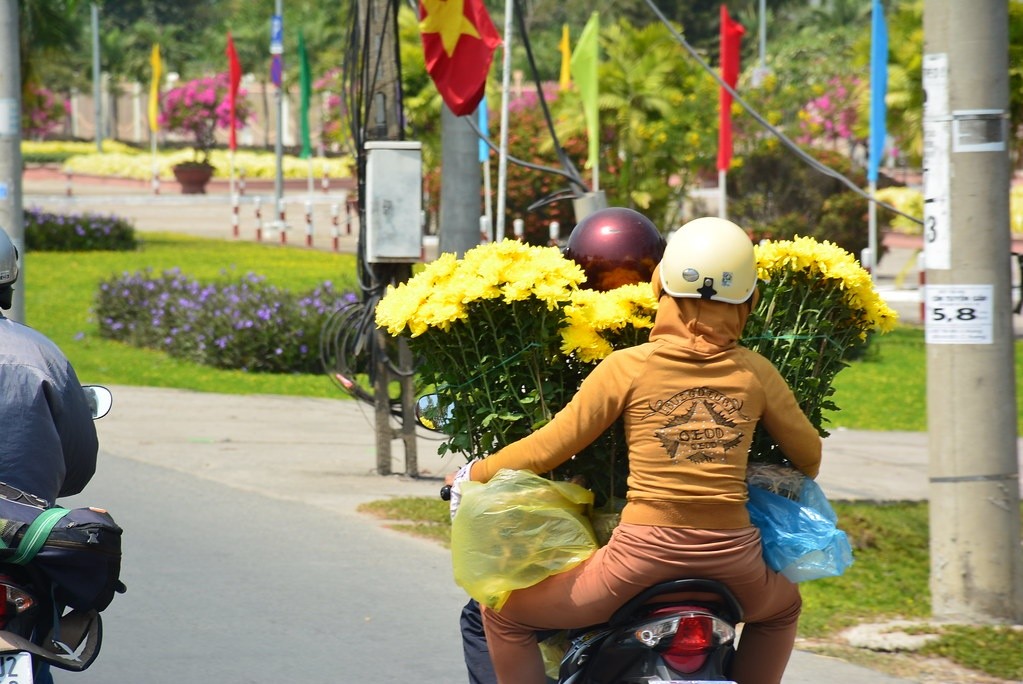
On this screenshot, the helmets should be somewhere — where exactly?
[565,208,667,292]
[659,217,758,304]
[0,226,19,286]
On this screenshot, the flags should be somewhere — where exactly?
[148,44,161,131]
[413,0,499,119]
[269,13,282,90]
[228,43,242,150]
[718,4,746,170]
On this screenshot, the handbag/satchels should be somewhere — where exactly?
[0,481,123,611]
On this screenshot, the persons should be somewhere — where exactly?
[453,215,823,684]
[458,207,666,683]
[0,228,99,684]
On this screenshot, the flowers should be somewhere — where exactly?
[558,279,657,505]
[373,239,587,461]
[736,235,900,503]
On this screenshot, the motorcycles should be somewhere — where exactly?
[441,481,747,683]
[0,385,112,684]
